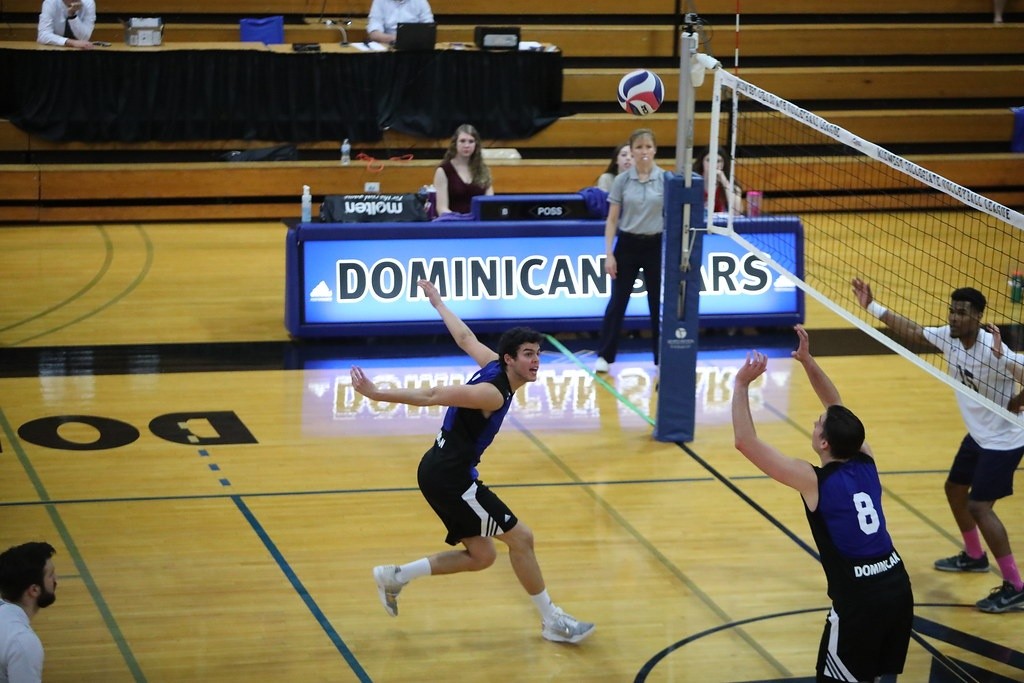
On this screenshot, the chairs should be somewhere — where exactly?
[239,16,284,44]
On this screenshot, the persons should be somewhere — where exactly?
[367,0,434,42]
[693,143,742,216]
[597,143,637,192]
[850,273,1024,613]
[0,542,57,683]
[349,280,595,645]
[37,0,96,48]
[434,124,494,217]
[598,129,665,372]
[731,324,914,683]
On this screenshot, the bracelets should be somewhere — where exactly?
[999,354,1013,369]
[865,300,888,320]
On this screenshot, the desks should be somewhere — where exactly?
[0,41,563,143]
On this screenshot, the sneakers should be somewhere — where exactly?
[934,551,989,573]
[541,608,595,644]
[372,565,405,618]
[976,580,1024,613]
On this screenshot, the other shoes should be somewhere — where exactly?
[595,357,608,372]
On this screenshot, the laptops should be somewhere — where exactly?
[392,23,437,49]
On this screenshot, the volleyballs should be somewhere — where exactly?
[616,68,666,116]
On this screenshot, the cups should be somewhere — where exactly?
[747,192,762,217]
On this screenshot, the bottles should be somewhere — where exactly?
[340,139,350,165]
[1011,272,1022,302]
[418,184,437,219]
[302,185,311,222]
